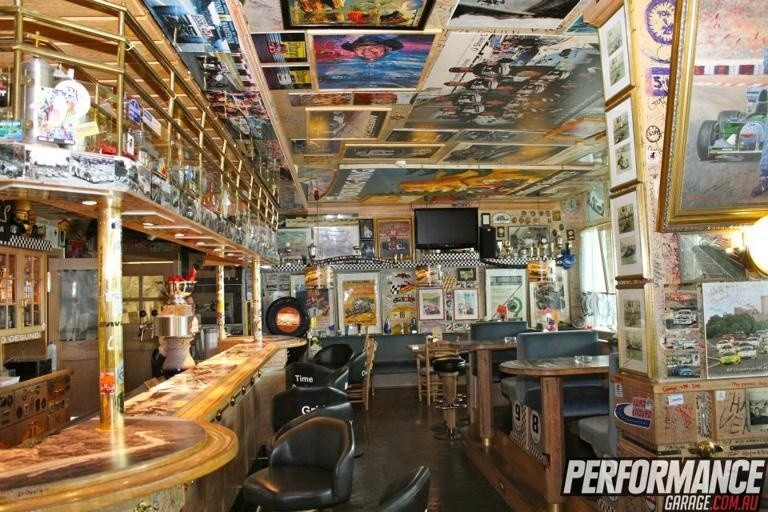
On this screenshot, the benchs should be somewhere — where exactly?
[471,321,528,406]
[502,330,609,465]
[579,353,619,457]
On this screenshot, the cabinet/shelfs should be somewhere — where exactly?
[0,246,47,336]
[0,0,281,267]
[0,367,73,451]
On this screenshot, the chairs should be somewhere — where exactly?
[242,334,431,512]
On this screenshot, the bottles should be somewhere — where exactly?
[384,316,418,336]
[497,305,506,322]
[352,246,361,256]
[47,339,57,370]
[329,320,335,336]
[497,236,556,259]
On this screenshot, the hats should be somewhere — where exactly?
[342,35,403,51]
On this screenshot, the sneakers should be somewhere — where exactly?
[753,180,768,195]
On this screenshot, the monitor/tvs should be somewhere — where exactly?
[414,207,478,249]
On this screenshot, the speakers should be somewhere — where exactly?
[480,225,496,258]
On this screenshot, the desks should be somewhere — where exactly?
[469,342,517,445]
[499,355,609,474]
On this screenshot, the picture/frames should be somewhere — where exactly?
[289,213,571,335]
[598,3,768,378]
[250,0,606,206]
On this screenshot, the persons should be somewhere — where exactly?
[434,57,592,122]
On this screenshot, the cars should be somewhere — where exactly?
[750,398,767,420]
[660,301,768,381]
[269,41,288,55]
[277,73,295,85]
[695,87,768,166]
[472,37,593,128]
